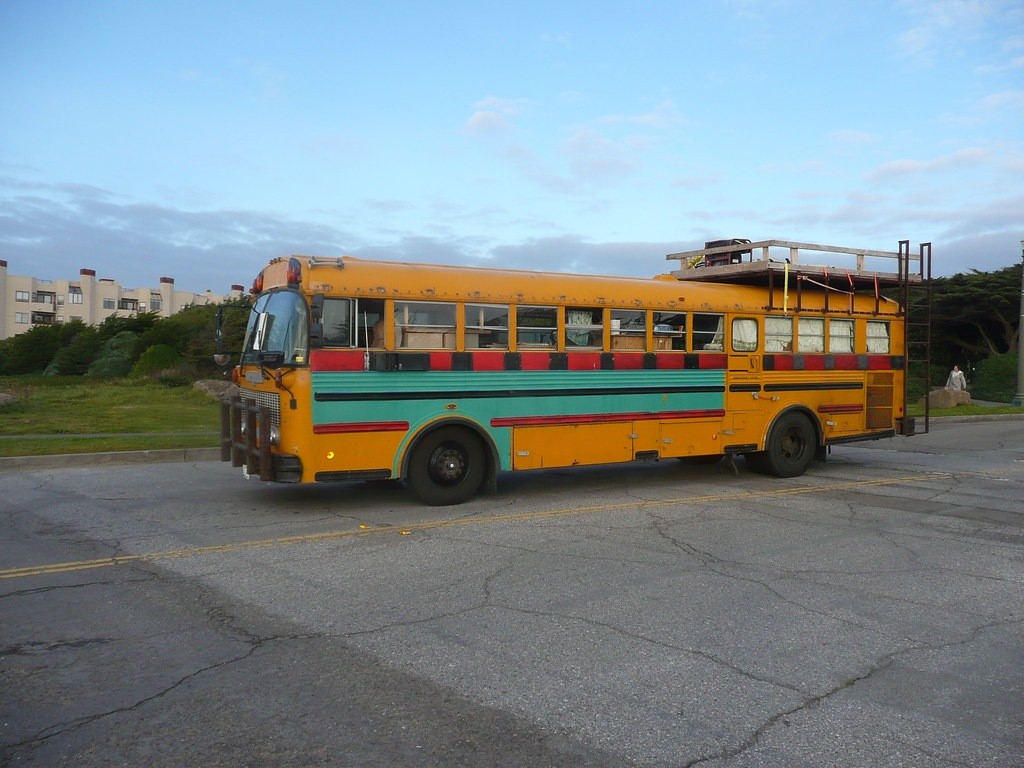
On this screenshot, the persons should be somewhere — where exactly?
[945,365,967,391]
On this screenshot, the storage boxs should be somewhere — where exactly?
[609,319,620,335]
[403,332,479,348]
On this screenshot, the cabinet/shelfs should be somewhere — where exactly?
[592,326,683,350]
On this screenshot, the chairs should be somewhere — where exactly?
[370,318,402,348]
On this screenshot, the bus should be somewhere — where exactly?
[213,238,934,509]
